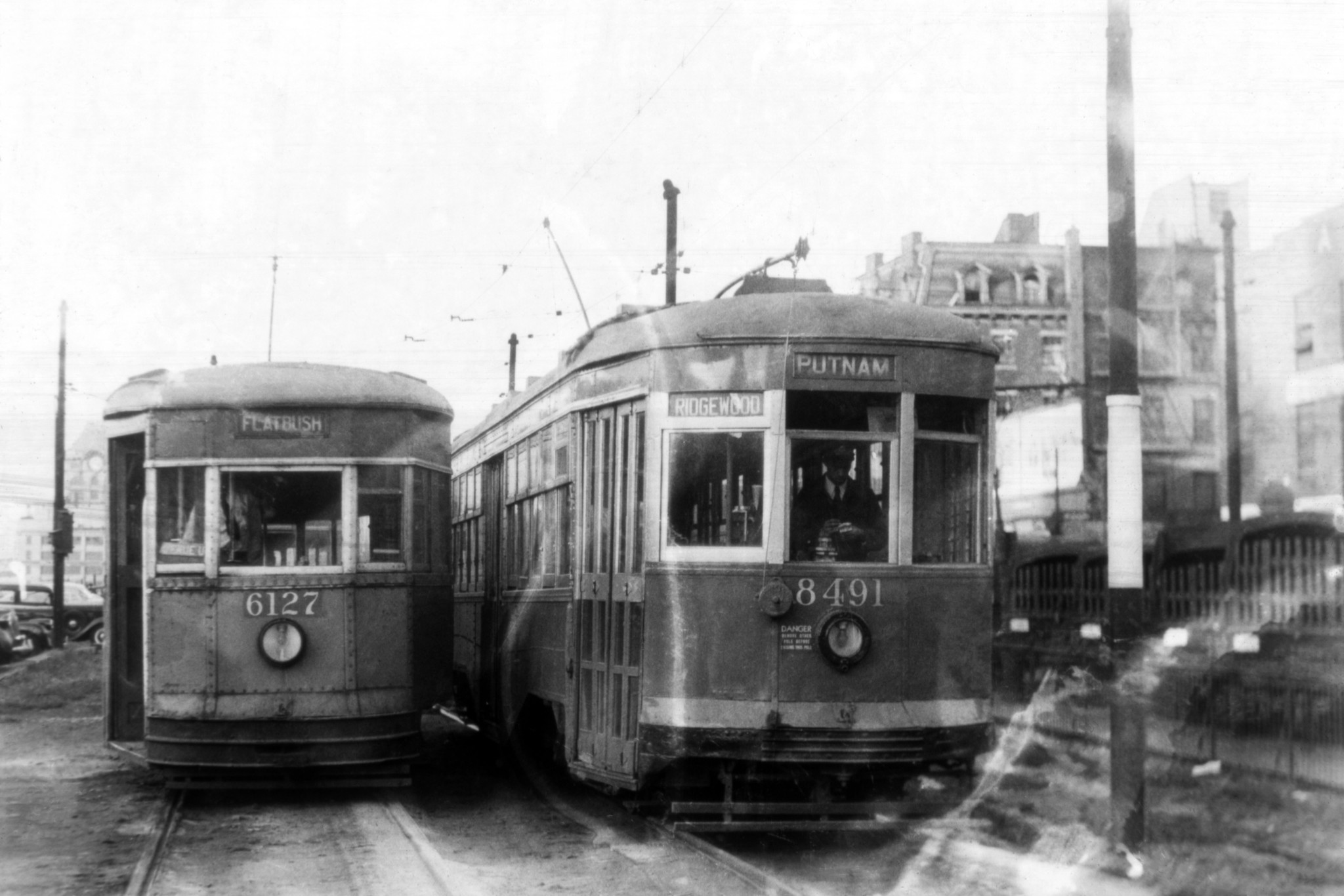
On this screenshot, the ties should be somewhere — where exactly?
[834,487,843,509]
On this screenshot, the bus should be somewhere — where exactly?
[449,176,1015,833]
[99,254,456,797]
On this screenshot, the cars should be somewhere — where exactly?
[0,581,105,664]
[994,516,1225,604]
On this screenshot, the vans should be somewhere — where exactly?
[1216,513,1344,621]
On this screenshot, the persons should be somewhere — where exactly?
[183,489,249,563]
[790,443,888,561]
